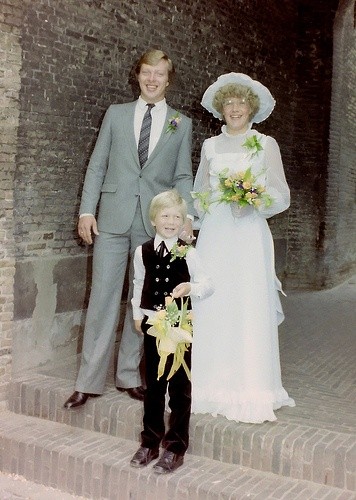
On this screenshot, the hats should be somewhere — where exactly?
[201,72,275,123]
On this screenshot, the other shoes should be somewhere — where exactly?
[130,443,159,465]
[153,450,185,472]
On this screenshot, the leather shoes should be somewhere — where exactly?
[116,386,145,402]
[64,391,101,410]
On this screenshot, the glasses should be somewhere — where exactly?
[222,98,249,109]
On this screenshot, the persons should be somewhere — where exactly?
[164,71,296,424]
[64,50,200,408]
[131,189,216,474]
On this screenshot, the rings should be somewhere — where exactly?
[187,235,193,239]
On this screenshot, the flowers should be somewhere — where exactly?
[170,243,189,262]
[242,136,264,157]
[146,296,193,380]
[165,113,180,135]
[191,166,273,215]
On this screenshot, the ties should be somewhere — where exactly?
[138,103,155,170]
[157,241,166,257]
